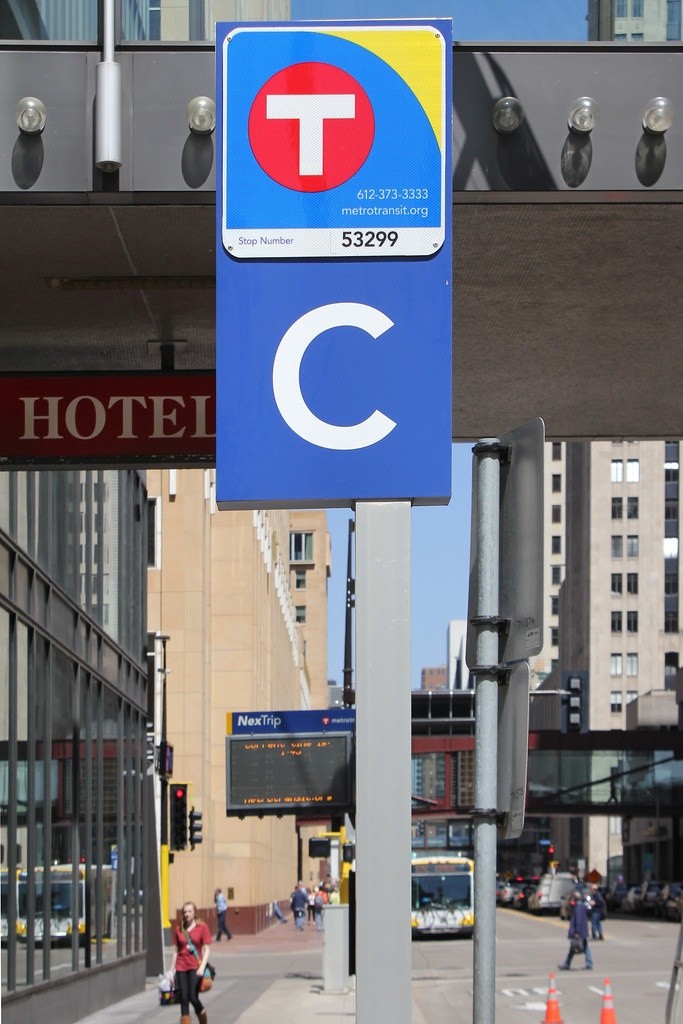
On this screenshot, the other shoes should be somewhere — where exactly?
[227,936,232,941]
[300,925,304,931]
[558,966,569,970]
[581,966,591,970]
[591,938,597,941]
[598,936,603,940]
[280,919,288,924]
[214,939,220,942]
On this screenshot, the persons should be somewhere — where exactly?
[558,892,592,971]
[590,885,603,941]
[290,882,339,931]
[608,781,617,803]
[170,902,212,1024]
[274,901,288,924]
[215,888,232,942]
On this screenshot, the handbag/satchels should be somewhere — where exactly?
[600,910,607,921]
[157,973,179,1006]
[200,963,216,992]
[571,935,587,954]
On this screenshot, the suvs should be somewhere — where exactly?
[526,873,577,916]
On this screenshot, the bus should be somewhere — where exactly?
[1,859,113,948]
[410,856,474,939]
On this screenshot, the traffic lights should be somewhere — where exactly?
[169,783,187,850]
[189,805,203,850]
[549,845,554,854]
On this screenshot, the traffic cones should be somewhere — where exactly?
[541,973,565,1024]
[599,978,618,1024]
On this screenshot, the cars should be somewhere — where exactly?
[621,880,683,919]
[496,876,539,910]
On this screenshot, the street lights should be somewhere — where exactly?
[156,636,173,946]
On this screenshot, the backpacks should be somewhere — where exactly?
[314,892,325,913]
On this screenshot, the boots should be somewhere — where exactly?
[181,1015,191,1024]
[197,1011,207,1024]
